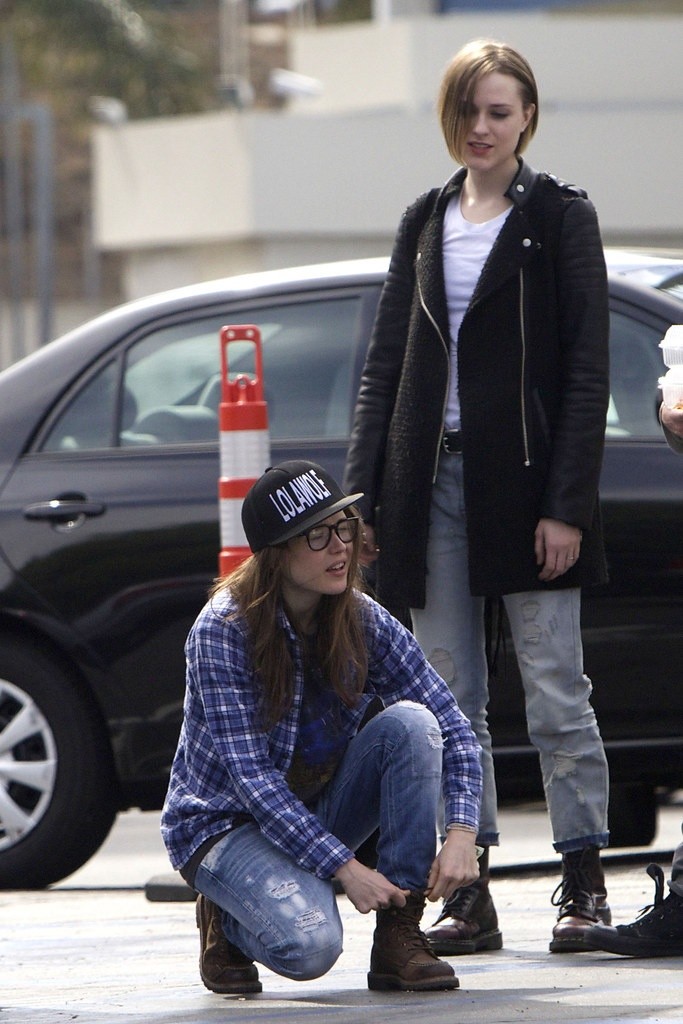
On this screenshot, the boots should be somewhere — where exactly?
[195,894,263,993]
[367,887,459,991]
[548,847,611,951]
[421,845,503,953]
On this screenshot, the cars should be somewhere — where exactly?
[0,243,683,897]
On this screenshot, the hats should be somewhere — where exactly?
[241,460,364,552]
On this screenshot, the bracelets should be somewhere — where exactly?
[445,825,479,835]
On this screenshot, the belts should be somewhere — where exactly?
[440,428,463,454]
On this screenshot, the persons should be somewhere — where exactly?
[587,262,682,960]
[346,37,612,953]
[157,458,484,998]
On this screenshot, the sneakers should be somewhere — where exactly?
[583,862,683,958]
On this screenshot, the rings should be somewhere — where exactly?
[566,556,574,560]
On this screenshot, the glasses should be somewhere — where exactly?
[296,516,358,551]
